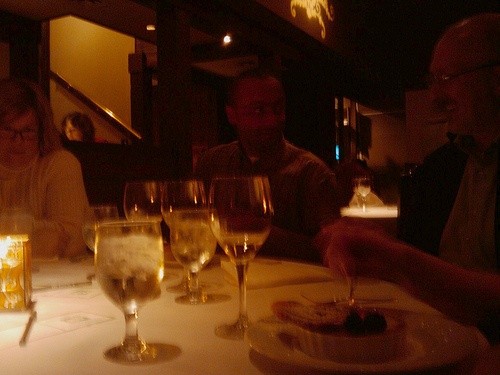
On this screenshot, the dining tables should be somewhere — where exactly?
[2,252,477,374]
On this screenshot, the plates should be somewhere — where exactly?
[246,303,476,375]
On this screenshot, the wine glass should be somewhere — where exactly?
[168,208,220,303]
[93,219,165,365]
[80,205,121,282]
[208,176,275,340]
[162,178,214,271]
[123,180,168,283]
[353,176,372,215]
[2,206,40,274]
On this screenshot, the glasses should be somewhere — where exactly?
[0,126,43,141]
[423,61,499,90]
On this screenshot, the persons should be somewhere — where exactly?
[397,132,475,248]
[307,10,500,327]
[193,67,340,258]
[0,77,100,259]
[58,113,94,142]
